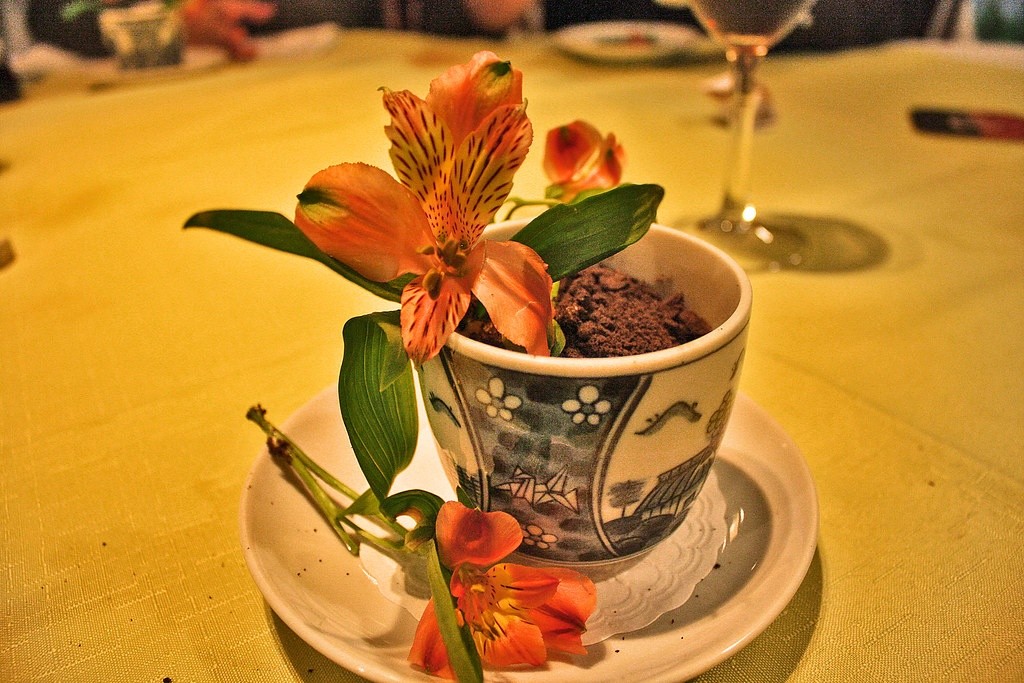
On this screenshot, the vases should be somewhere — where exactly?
[402,218,751,579]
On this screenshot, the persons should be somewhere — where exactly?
[26,0,543,62]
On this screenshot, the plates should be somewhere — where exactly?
[71,48,225,86]
[550,21,704,62]
[242,374,818,683]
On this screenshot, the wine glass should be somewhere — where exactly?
[667,0,818,267]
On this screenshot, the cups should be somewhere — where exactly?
[96,8,189,72]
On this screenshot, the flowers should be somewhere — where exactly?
[183,43,665,683]
